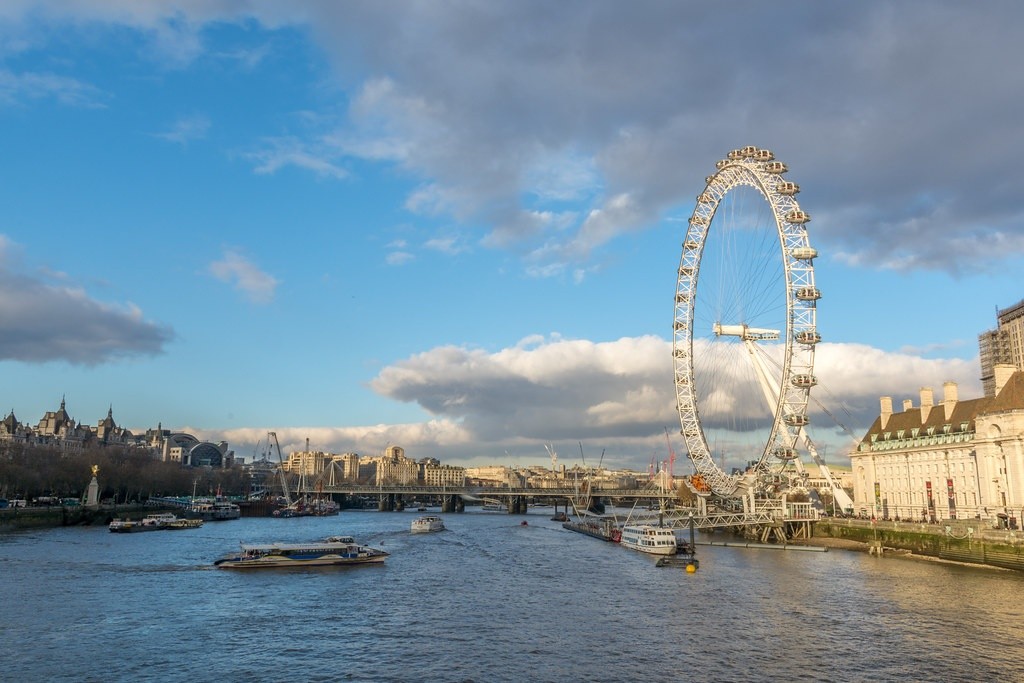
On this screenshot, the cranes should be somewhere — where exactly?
[648,425,677,490]
[264,432,299,516]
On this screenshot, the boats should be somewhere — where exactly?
[410,515,445,536]
[213,535,391,568]
[619,524,678,555]
[108,512,204,533]
